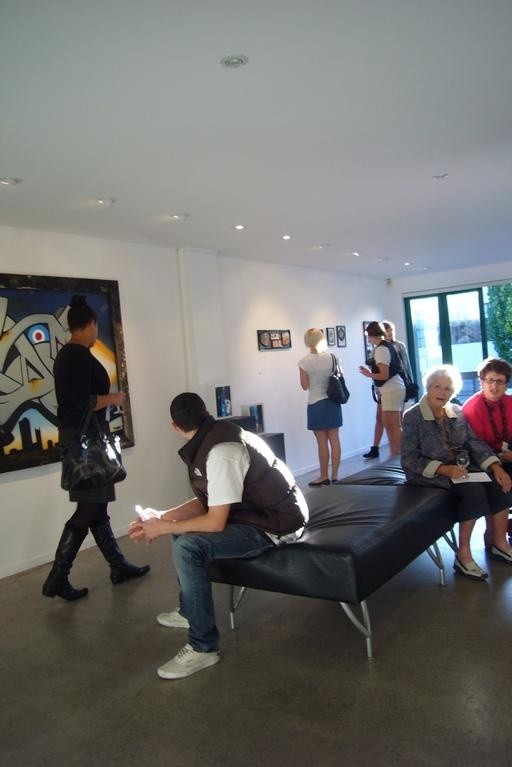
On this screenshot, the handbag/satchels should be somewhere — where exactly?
[61,407,126,489]
[327,354,349,403]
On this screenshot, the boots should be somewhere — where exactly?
[92,522,150,583]
[363,447,379,458]
[42,528,88,599]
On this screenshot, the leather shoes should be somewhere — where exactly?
[454,556,488,580]
[490,544,512,563]
[309,479,330,486]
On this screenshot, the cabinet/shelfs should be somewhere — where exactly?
[225,414,286,465]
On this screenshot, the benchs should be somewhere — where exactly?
[207,484,454,660]
[335,453,459,554]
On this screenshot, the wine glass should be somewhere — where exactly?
[456,453,471,479]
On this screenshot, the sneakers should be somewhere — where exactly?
[157,643,221,680]
[157,608,191,628]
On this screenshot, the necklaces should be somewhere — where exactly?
[484,395,508,448]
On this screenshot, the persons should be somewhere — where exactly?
[462,359,511,549]
[42,296,149,601]
[400,366,512,578]
[298,328,342,485]
[127,393,308,679]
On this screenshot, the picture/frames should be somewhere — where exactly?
[0,272,135,474]
[336,326,346,347]
[363,321,374,365]
[326,327,336,346]
[257,329,292,351]
